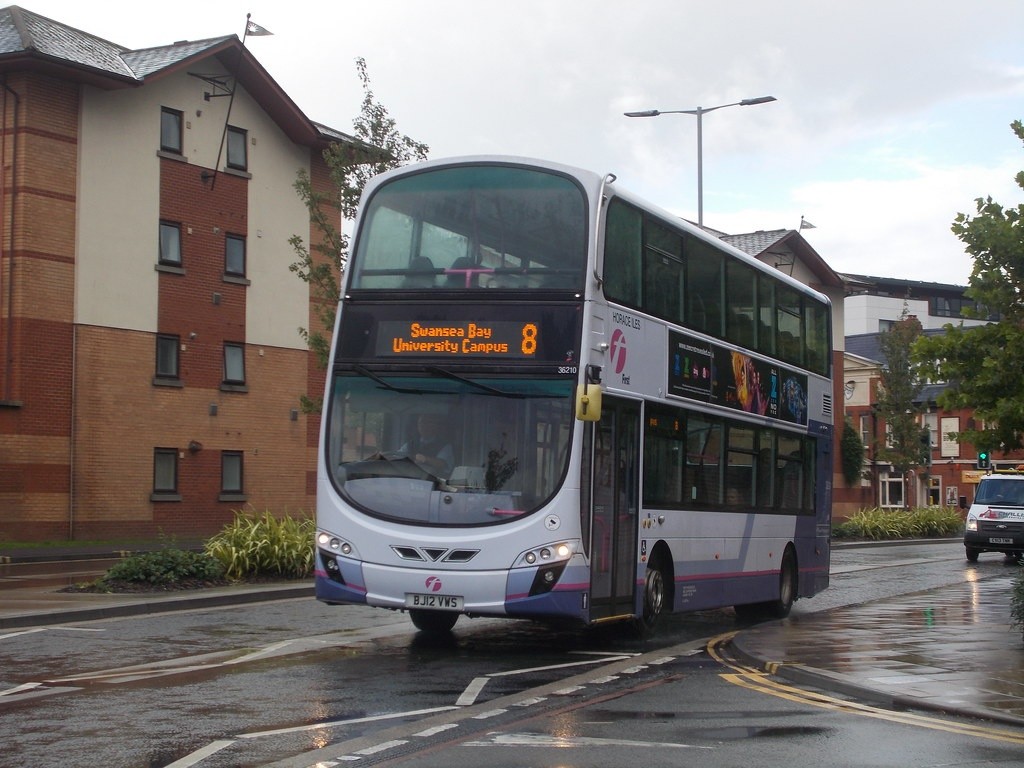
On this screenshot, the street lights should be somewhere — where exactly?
[621,95,779,235]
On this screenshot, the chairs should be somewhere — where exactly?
[643,267,830,377]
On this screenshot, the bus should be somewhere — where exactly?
[309,153,834,636]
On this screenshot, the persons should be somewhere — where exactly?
[392,414,455,477]
[399,243,827,375]
[726,489,738,504]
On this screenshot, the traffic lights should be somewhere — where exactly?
[977,449,991,469]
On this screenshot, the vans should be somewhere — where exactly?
[957,473,1024,560]
